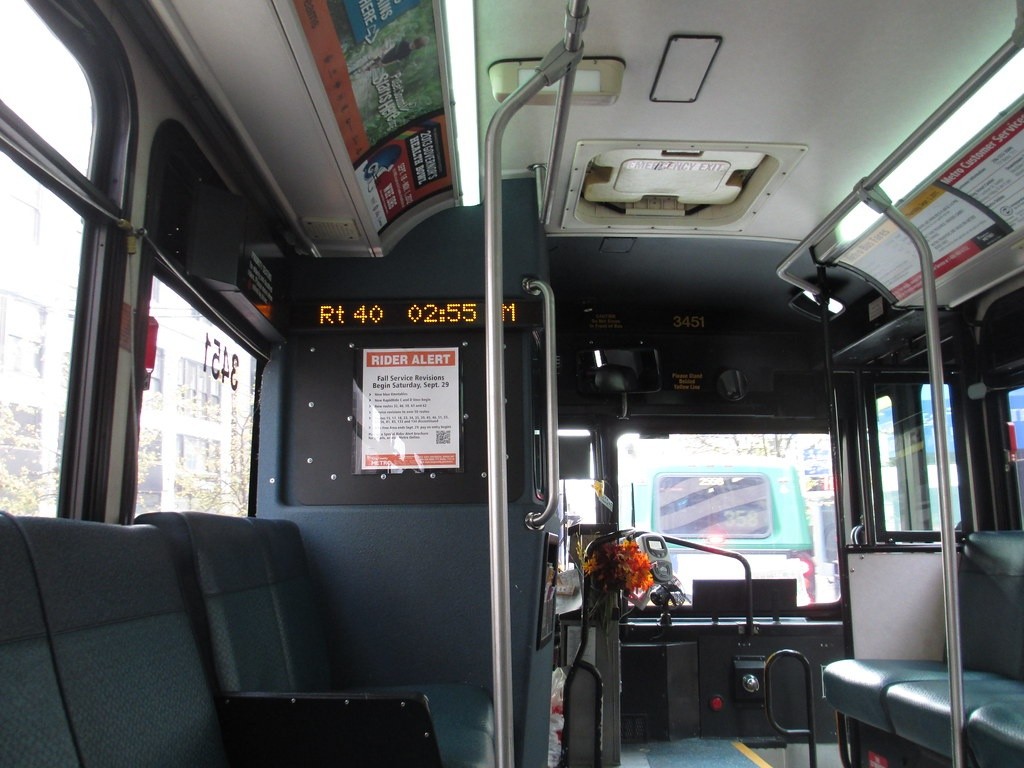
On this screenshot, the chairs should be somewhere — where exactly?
[821,531,1024,741]
[884,674,1024,767]
[138,510,498,768]
[15,518,233,768]
[967,702,1024,768]
[247,516,497,743]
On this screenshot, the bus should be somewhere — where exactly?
[633,459,819,611]
